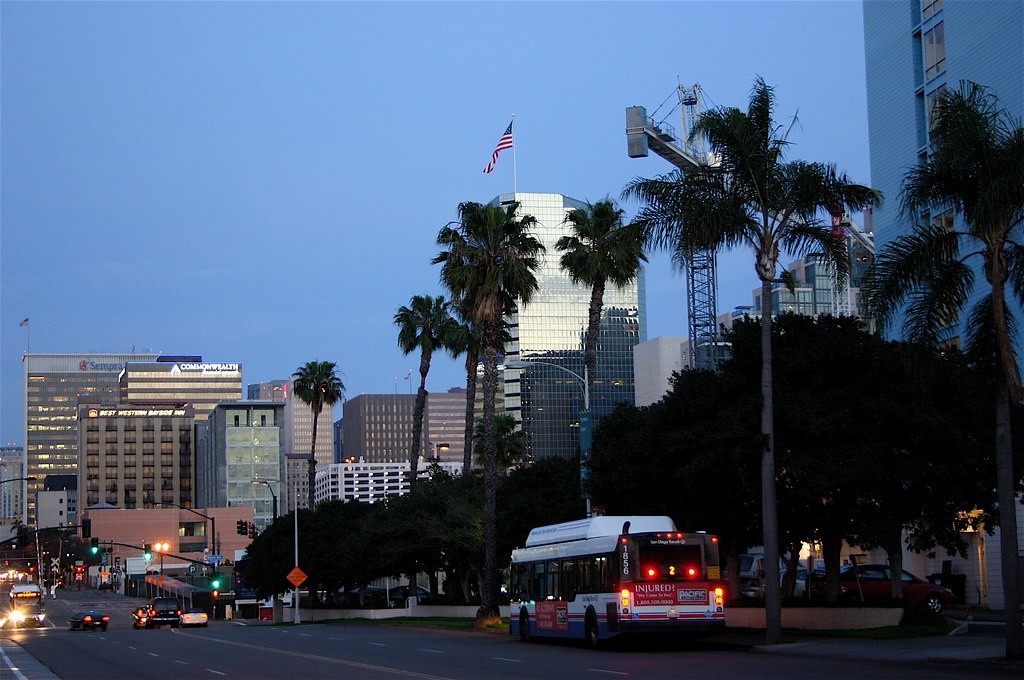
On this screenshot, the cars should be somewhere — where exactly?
[719,553,825,608]
[323,581,433,609]
[68,609,108,633]
[808,563,957,617]
[131,604,155,629]
[179,608,208,626]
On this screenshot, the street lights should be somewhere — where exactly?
[251,478,278,526]
[152,501,219,620]
[505,360,592,517]
[156,542,169,597]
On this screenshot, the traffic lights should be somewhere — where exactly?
[248,524,255,539]
[236,520,243,535]
[209,571,221,601]
[234,571,242,586]
[144,543,152,561]
[241,521,248,535]
[100,546,113,555]
[91,537,99,557]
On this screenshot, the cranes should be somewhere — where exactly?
[827,206,875,320]
[624,75,808,375]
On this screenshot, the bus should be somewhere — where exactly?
[7,584,47,628]
[508,506,727,651]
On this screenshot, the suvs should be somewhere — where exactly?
[147,597,181,629]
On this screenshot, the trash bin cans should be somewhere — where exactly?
[283,608,295,623]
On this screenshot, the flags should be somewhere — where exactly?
[480,119,513,174]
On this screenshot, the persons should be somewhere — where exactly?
[760,570,766,588]
